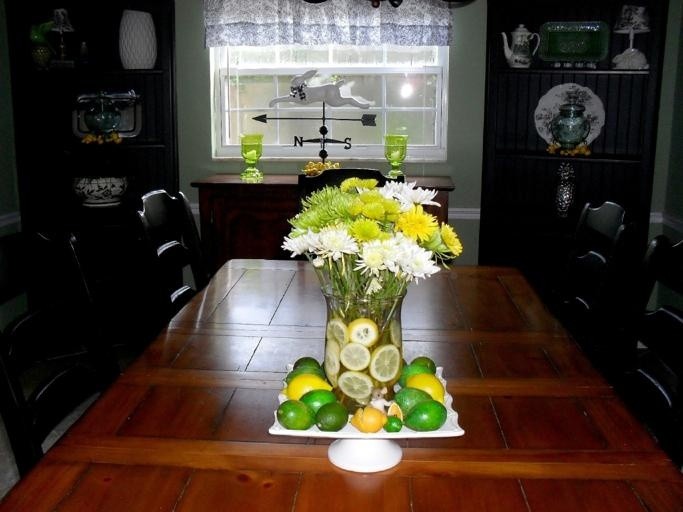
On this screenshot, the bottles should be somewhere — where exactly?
[547,94,591,150]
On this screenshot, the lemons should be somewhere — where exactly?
[277,318,446,433]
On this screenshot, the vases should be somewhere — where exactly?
[384,135,409,179]
[319,285,406,416]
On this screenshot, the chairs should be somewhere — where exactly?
[137,190,211,286]
[580,320,681,449]
[1,231,204,305]
[2,309,123,473]
[539,201,680,320]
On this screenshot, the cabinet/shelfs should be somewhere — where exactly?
[8,0,179,229]
[479,2,670,266]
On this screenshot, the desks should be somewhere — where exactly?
[194,174,456,281]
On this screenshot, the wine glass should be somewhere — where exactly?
[382,134,411,180]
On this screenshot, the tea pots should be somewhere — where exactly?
[501,24,540,69]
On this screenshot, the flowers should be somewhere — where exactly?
[284,176,464,341]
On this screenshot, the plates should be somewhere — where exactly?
[534,83,606,147]
